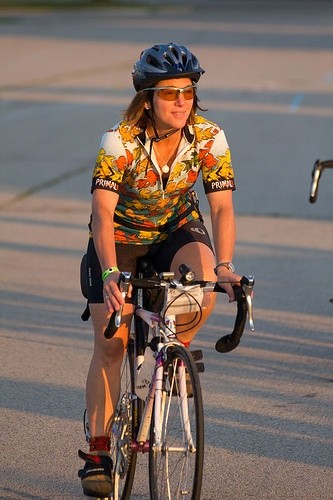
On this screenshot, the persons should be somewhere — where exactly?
[78,43,253,497]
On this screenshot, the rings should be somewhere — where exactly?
[103,296,109,300]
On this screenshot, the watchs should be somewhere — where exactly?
[214,261,235,275]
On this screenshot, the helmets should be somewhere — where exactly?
[132,43,205,93]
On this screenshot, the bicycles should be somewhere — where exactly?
[104,253,257,500]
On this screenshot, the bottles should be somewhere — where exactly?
[134,353,144,380]
[135,337,164,400]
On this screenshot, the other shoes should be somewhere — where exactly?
[168,350,205,398]
[77,448,114,498]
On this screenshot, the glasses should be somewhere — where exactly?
[143,85,197,101]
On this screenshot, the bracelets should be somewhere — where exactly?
[102,266,118,281]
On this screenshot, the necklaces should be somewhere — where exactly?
[147,129,180,174]
